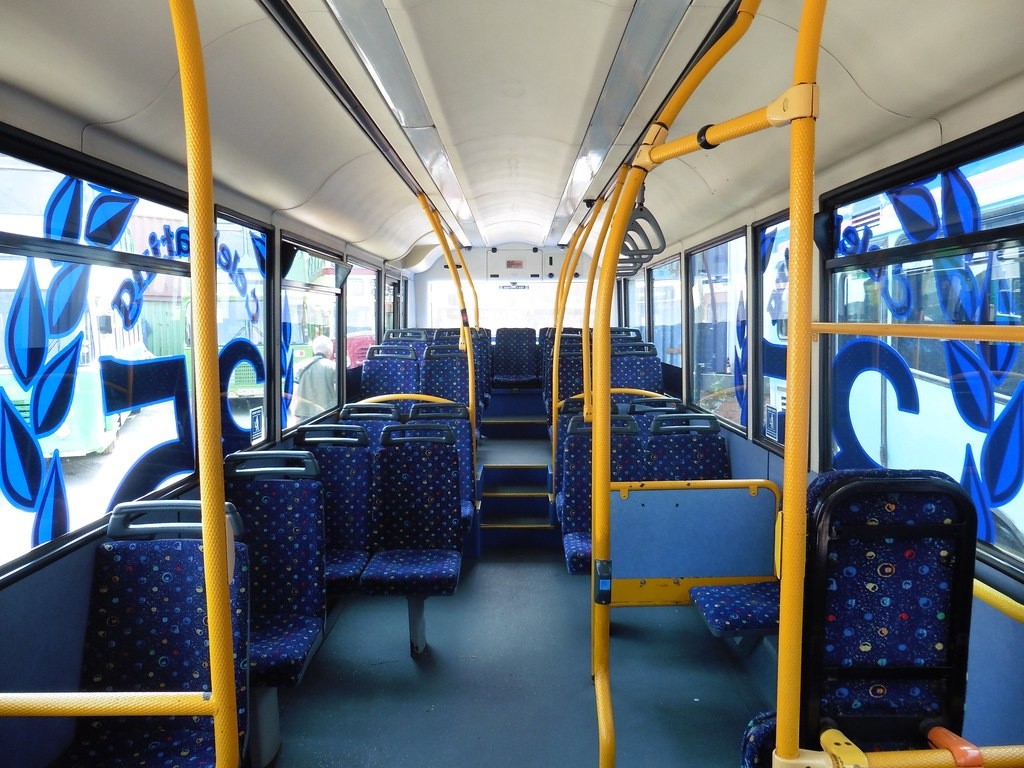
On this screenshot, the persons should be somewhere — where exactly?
[294,335,337,421]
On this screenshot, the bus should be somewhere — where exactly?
[725,161,1024,550]
[0,258,380,459]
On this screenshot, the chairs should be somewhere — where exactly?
[55,327,977,768]
